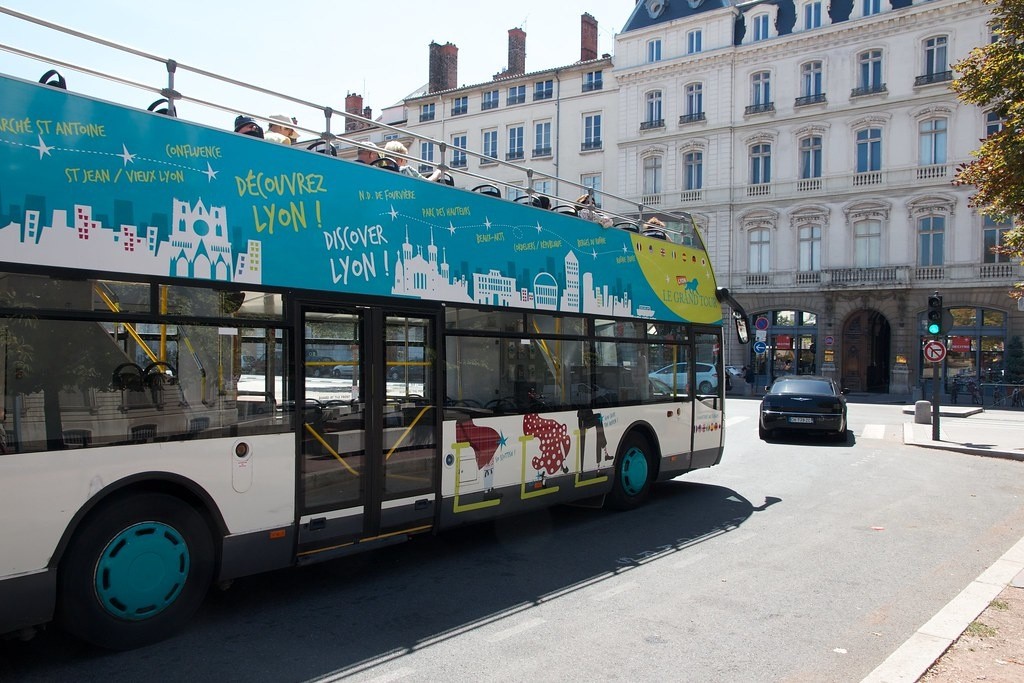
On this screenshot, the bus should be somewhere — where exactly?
[0,6,753,655]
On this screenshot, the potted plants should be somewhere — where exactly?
[1005,335,1024,398]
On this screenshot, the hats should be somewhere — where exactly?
[269,115,300,139]
[234,115,260,132]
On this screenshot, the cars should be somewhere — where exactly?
[759,375,851,443]
[241,355,255,371]
[250,351,282,376]
[305,356,337,378]
[647,363,743,395]
[333,358,359,378]
[389,359,423,381]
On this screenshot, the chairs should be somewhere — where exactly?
[113,360,526,454]
[37,67,668,241]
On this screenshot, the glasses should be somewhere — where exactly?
[246,124,259,133]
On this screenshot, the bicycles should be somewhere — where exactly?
[949,376,1024,408]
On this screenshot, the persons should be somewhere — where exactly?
[235,115,263,138]
[383,140,441,182]
[571,194,613,229]
[263,114,294,146]
[354,141,379,167]
[742,365,754,394]
[533,196,551,211]
[643,217,673,241]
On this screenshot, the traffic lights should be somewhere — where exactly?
[928,295,943,335]
